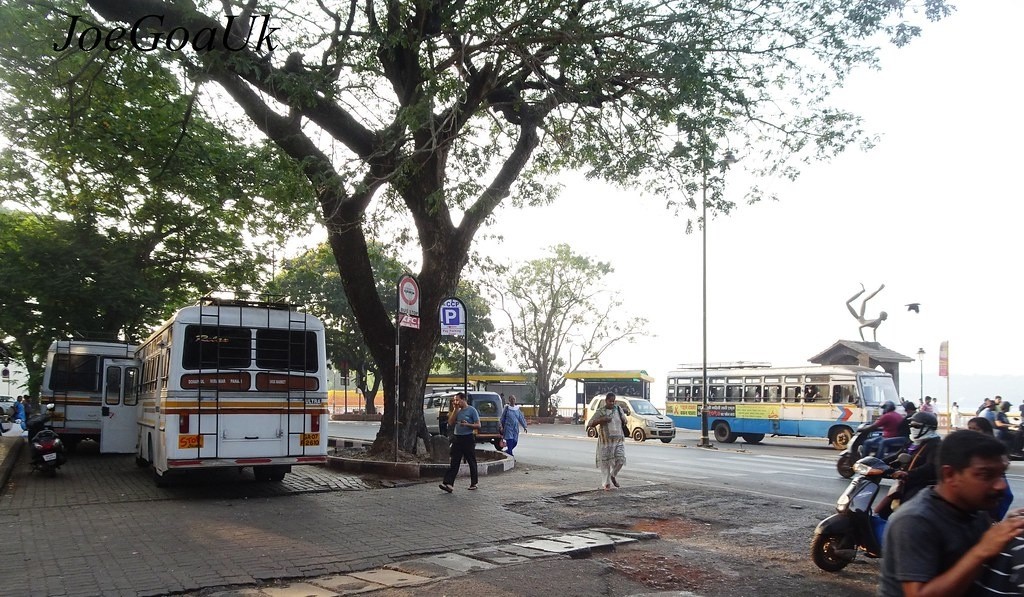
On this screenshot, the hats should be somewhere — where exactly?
[984,400,998,408]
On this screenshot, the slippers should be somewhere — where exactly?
[468,483,478,490]
[439,484,453,493]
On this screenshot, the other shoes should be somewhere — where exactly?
[611,475,620,488]
[605,484,611,491]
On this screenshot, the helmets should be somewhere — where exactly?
[908,412,938,427]
[1000,401,1012,409]
[881,401,896,410]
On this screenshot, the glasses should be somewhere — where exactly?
[909,423,922,429]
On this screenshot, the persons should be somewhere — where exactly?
[0,423,10,437]
[12,395,32,431]
[900,396,939,420]
[589,393,629,492]
[963,515,1024,597]
[860,401,1014,559]
[675,386,858,402]
[976,395,1024,459]
[439,393,482,493]
[951,402,961,431]
[500,392,506,407]
[875,430,1024,597]
[500,395,527,454]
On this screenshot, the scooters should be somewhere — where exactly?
[809,445,913,573]
[835,423,883,479]
[1003,417,1024,462]
[16,402,67,478]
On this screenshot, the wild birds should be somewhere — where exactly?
[905,303,920,313]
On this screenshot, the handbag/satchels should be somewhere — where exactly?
[500,439,507,447]
[498,406,508,436]
[617,405,630,437]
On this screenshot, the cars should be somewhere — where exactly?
[585,395,676,443]
[0,395,17,416]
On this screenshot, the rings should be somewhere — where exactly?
[992,521,1001,526]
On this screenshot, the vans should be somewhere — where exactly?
[423,387,505,451]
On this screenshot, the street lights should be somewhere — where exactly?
[916,347,926,406]
[697,117,737,448]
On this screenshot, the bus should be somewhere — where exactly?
[40,337,140,455]
[665,360,907,450]
[98,297,330,489]
